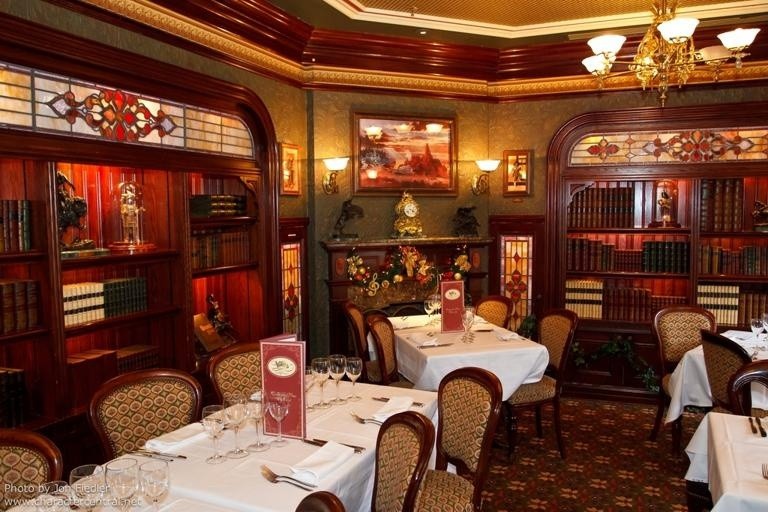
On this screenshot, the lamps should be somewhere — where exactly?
[581,0,764,109]
[470,158,500,198]
[320,154,351,196]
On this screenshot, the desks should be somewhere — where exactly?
[675,409,767,512]
[671,330,767,447]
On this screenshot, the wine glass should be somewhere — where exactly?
[304,353,362,418]
[425,293,442,328]
[36,458,172,511]
[749,313,768,354]
[459,304,476,344]
[201,388,290,465]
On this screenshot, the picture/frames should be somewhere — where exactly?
[347,104,462,201]
[277,140,305,200]
[502,147,535,199]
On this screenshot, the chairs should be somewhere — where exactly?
[645,300,718,451]
[86,367,208,464]
[723,357,767,422]
[367,413,434,512]
[294,489,348,512]
[206,330,264,404]
[0,413,59,512]
[409,364,511,512]
[493,304,579,468]
[345,302,383,387]
[696,320,767,418]
[364,312,416,391]
[475,296,515,330]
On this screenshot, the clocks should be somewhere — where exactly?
[390,192,424,238]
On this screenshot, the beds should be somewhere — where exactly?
[379,312,553,458]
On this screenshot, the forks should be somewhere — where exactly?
[260,464,316,487]
[349,411,386,430]
[761,462,768,480]
[260,469,312,492]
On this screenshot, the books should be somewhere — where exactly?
[0,190,47,339]
[58,275,160,410]
[186,187,251,272]
[0,362,28,432]
[565,179,768,328]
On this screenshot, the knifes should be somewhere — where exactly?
[476,329,497,333]
[126,445,187,463]
[417,342,452,350]
[303,437,366,456]
[372,395,427,409]
[748,415,768,438]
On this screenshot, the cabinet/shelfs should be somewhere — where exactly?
[557,164,767,400]
[36,152,192,463]
[172,168,272,403]
[0,144,61,482]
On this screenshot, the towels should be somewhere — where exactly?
[374,394,413,425]
[139,418,204,452]
[292,440,353,479]
